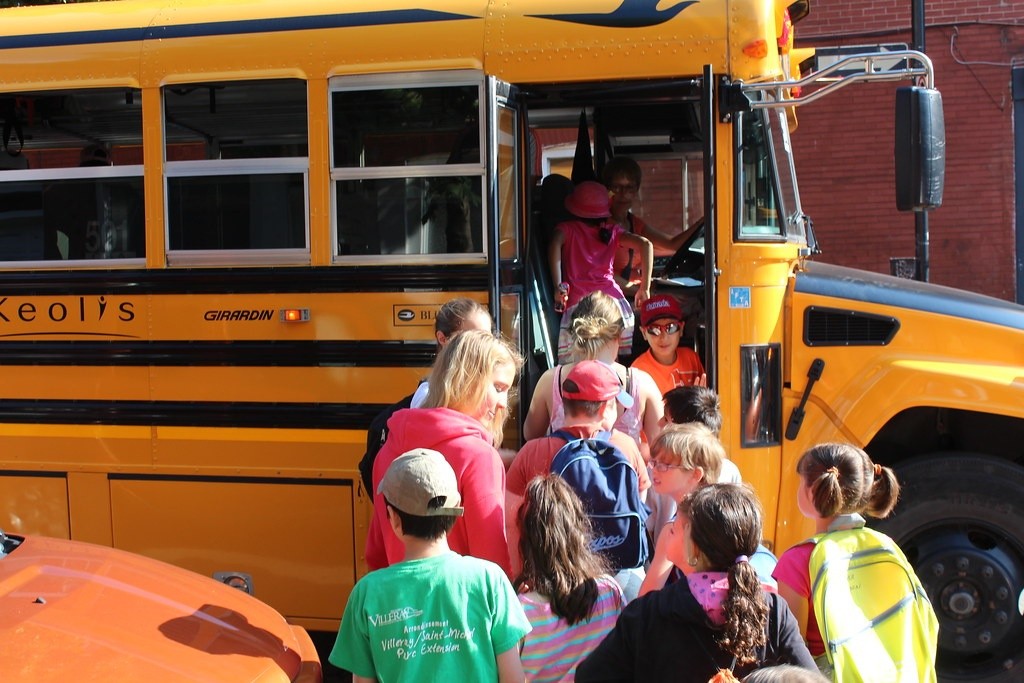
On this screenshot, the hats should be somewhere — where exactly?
[562,360,634,410]
[564,180,611,218]
[641,293,681,329]
[377,447,464,517]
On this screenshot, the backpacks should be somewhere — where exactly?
[789,526,939,683]
[549,426,654,569]
[359,378,431,505]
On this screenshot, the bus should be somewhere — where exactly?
[0,0,1024,682]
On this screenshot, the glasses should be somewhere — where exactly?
[648,457,691,472]
[645,321,682,336]
[607,183,638,194]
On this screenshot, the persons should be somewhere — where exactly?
[710,665,830,683]
[505,361,653,579]
[650,424,724,500]
[605,156,705,315]
[329,449,532,683]
[410,297,491,408]
[644,385,742,552]
[549,181,655,360]
[513,473,628,683]
[523,292,666,466]
[666,494,696,576]
[769,443,899,673]
[366,331,521,583]
[573,483,818,683]
[631,293,707,442]
[0,132,480,261]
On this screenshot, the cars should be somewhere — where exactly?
[0,532,320,683]
